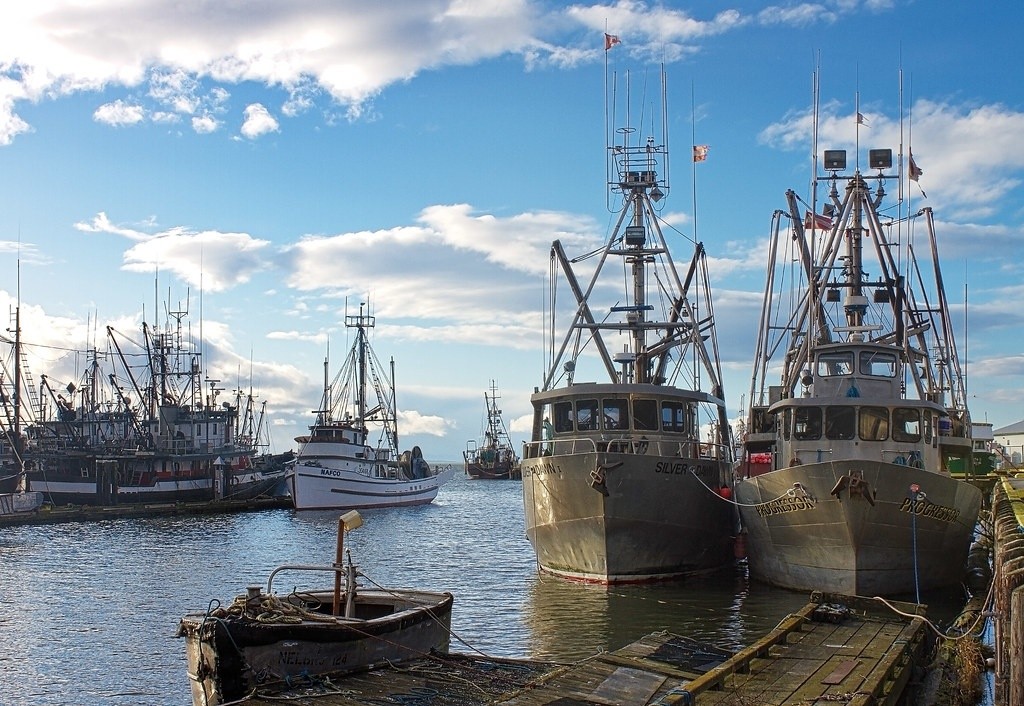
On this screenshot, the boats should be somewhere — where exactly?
[177,578,456,699]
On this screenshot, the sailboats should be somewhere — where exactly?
[0,228,293,533]
[520,14,740,582]
[461,377,522,480]
[288,298,449,512]
[923,281,999,500]
[736,42,983,598]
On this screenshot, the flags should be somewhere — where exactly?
[858,112,871,127]
[605,33,621,50]
[804,211,834,230]
[910,155,923,181]
[694,145,710,162]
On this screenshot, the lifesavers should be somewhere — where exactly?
[363,447,370,459]
[229,477,240,485]
